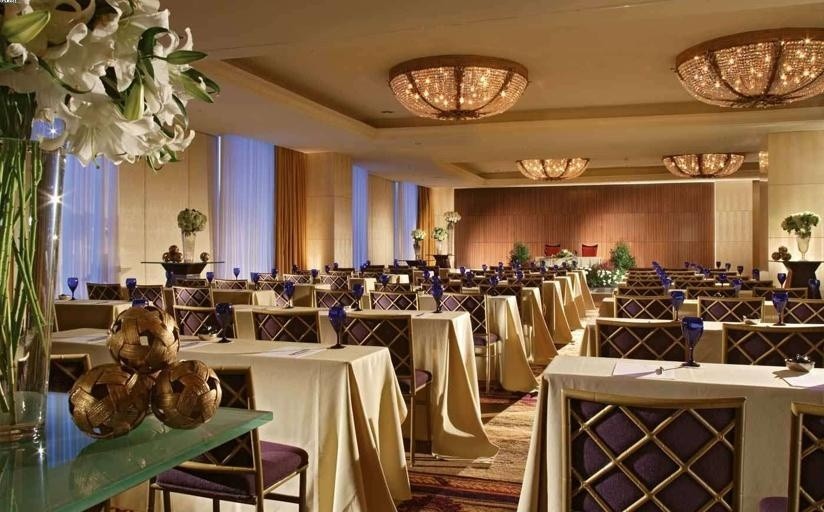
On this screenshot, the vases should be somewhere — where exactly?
[413,245,423,260]
[0,136,62,447]
[431,254,452,268]
[182,231,196,263]
[797,236,811,259]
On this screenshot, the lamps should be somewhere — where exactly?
[662,151,744,180]
[675,26,823,114]
[386,54,530,122]
[757,148,768,174]
[517,156,589,182]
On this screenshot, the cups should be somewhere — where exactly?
[205,272,215,284]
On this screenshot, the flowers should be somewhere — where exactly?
[431,212,462,255]
[780,211,819,238]
[176,208,207,237]
[0,0,222,177]
[410,229,425,244]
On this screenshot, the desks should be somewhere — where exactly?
[70,294,500,463]
[515,353,822,509]
[534,256,601,270]
[54,328,414,510]
[1,387,272,512]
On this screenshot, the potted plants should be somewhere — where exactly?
[608,236,636,284]
[509,240,532,269]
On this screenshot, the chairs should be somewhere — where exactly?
[146,366,308,512]
[582,264,823,368]
[52,263,595,391]
[50,352,93,389]
[341,313,434,468]
[543,243,562,256]
[755,400,824,510]
[582,244,598,257]
[559,385,747,510]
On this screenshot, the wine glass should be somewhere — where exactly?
[284,285,296,309]
[251,259,567,293]
[216,303,234,343]
[68,277,79,300]
[329,306,347,349]
[430,288,444,313]
[353,283,364,312]
[126,278,137,302]
[233,268,241,279]
[651,260,820,368]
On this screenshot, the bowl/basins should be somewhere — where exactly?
[58,294,71,300]
[785,358,815,372]
